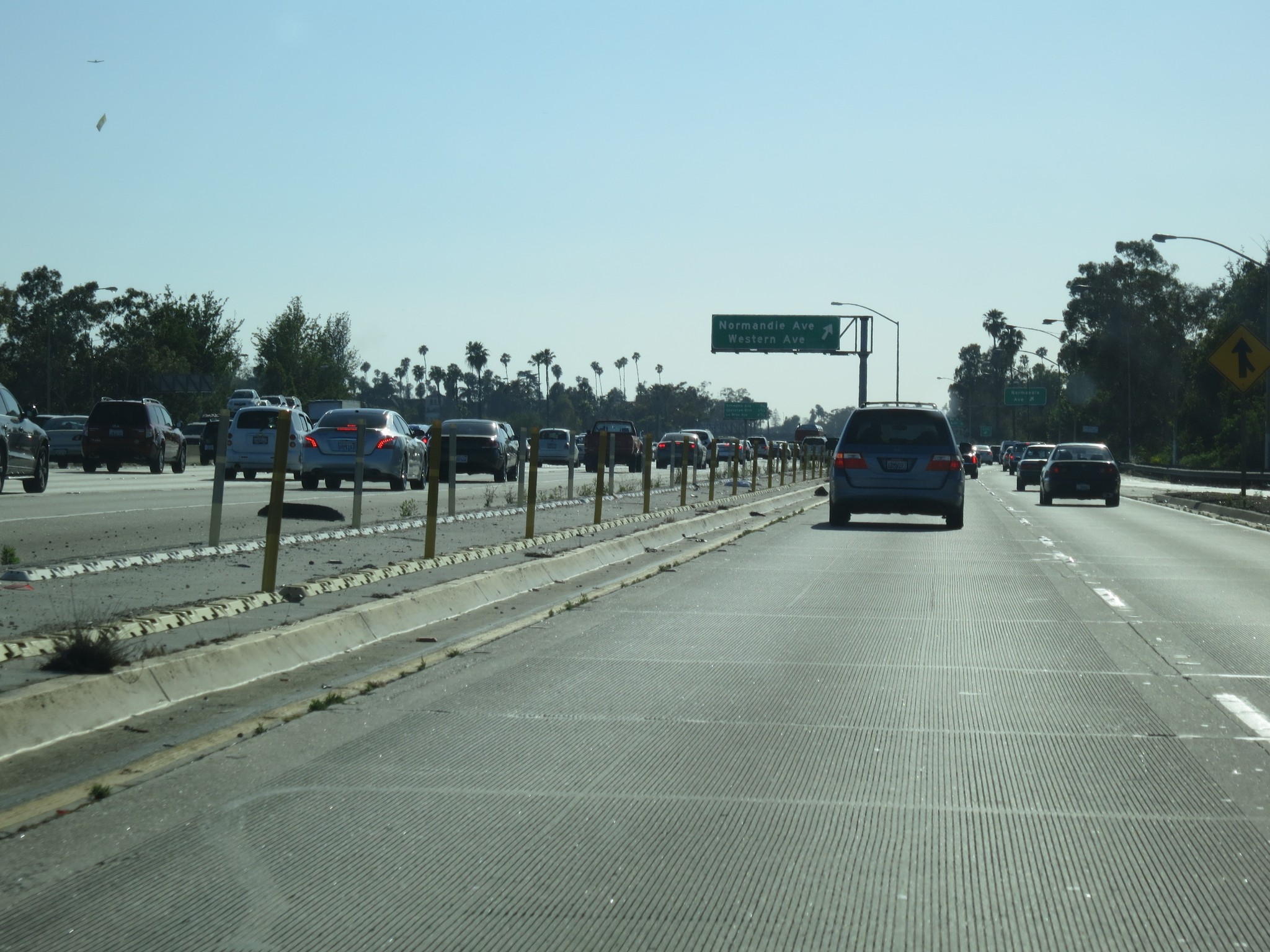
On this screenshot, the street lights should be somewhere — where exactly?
[982,361,1031,443]
[1003,324,1077,445]
[1041,317,1134,464]
[995,348,1062,444]
[830,301,899,407]
[936,376,971,443]
[1071,283,1177,468]
[1151,232,1270,474]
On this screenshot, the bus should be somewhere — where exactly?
[535,428,580,470]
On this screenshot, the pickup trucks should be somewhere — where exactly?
[583,420,643,473]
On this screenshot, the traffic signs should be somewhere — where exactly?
[711,314,841,351]
[1004,387,1047,406]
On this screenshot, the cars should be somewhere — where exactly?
[1014,443,1065,492]
[39,414,90,469]
[299,407,428,491]
[0,382,50,496]
[655,431,708,470]
[426,418,520,483]
[185,380,832,483]
[224,406,314,481]
[1039,441,1120,507]
[954,441,1047,480]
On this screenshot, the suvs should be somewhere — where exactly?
[824,401,973,530]
[80,396,188,474]
